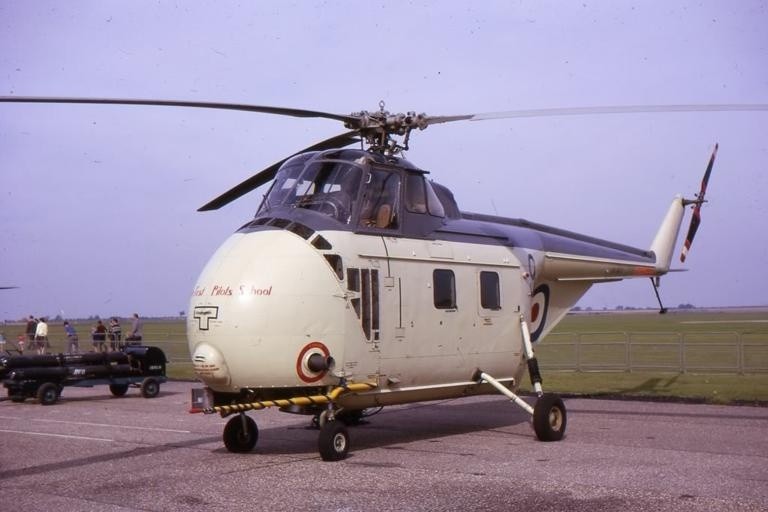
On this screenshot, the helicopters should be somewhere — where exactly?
[0,96,767,462]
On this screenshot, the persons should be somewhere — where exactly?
[26,313,142,354]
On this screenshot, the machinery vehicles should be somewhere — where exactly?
[1,333,169,405]
